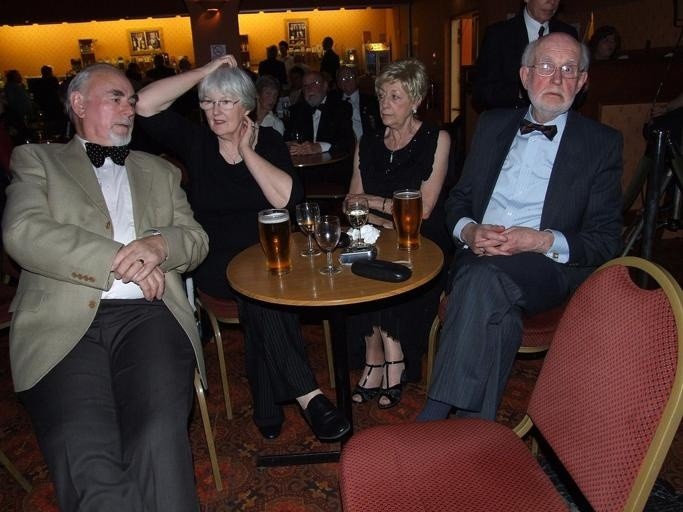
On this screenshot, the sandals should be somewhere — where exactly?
[353,361,385,403]
[378,360,408,409]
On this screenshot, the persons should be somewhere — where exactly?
[470,0,590,112]
[412,33,628,429]
[339,56,460,412]
[127,50,349,445]
[0,36,373,182]
[0,62,210,511]
[586,26,622,64]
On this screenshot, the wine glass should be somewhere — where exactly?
[346,195,371,247]
[296,201,322,258]
[312,215,343,276]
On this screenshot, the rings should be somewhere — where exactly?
[482,249,486,254]
[134,258,146,267]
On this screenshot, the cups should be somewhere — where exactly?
[290,130,303,145]
[257,208,294,277]
[392,188,423,252]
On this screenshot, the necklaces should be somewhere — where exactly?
[216,146,242,167]
[386,130,407,164]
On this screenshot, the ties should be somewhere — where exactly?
[539,26,544,37]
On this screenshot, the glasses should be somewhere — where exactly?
[528,62,579,79]
[303,80,321,89]
[199,99,239,110]
[341,76,355,80]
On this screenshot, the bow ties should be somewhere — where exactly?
[85,143,130,167]
[310,104,324,114]
[520,120,557,141]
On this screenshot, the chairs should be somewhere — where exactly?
[423,210,647,409]
[183,270,301,485]
[340,256,681,512]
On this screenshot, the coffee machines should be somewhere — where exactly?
[345,50,357,68]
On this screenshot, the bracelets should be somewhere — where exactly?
[381,195,389,214]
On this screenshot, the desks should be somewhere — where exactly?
[227,228,444,468]
[278,141,345,216]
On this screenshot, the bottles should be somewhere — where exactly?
[96,53,176,71]
[288,45,323,57]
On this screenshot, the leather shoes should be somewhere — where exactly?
[260,421,282,439]
[302,394,350,440]
